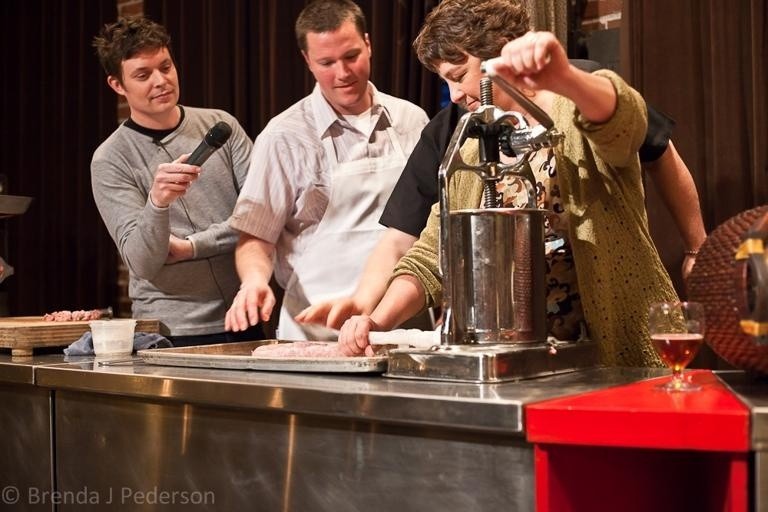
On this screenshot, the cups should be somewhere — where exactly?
[87,318,138,357]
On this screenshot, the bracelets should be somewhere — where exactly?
[684,249,699,256]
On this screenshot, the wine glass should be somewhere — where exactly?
[646,300,708,395]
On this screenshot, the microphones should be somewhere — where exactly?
[187,121,233,166]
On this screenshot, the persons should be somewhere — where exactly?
[291,58,713,332]
[338,0,680,370]
[89,16,269,349]
[223,0,434,339]
